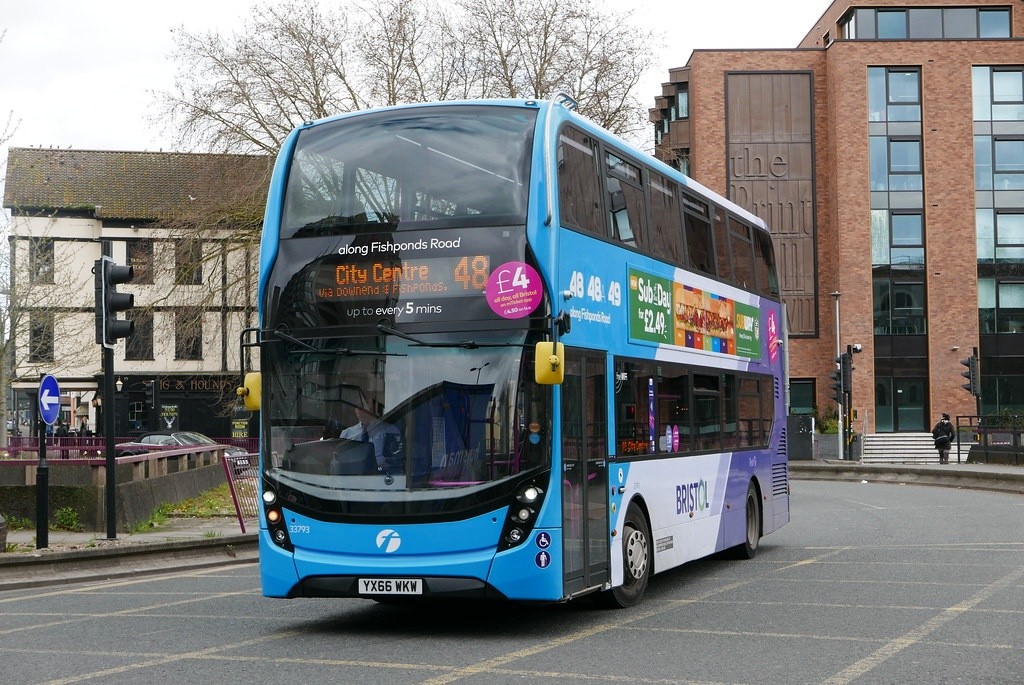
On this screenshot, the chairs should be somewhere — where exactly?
[692,251,711,274]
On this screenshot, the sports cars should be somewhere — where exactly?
[115,430,251,477]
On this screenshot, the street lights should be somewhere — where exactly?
[115,376,127,393]
[830,291,845,459]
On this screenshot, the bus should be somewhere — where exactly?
[236,92,789,610]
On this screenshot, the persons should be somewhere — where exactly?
[60,419,69,437]
[340,391,403,469]
[931,412,956,464]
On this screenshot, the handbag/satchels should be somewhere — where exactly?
[933,428,940,439]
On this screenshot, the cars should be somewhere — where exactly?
[7,421,23,447]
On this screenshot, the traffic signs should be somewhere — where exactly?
[37,375,61,425]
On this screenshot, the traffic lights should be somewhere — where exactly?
[835,352,852,393]
[91,255,134,350]
[829,372,843,405]
[960,355,980,396]
[145,375,160,411]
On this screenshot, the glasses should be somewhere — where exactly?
[355,402,370,408]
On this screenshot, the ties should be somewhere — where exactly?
[362,423,369,442]
